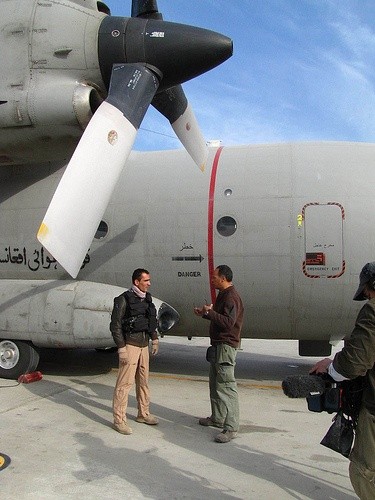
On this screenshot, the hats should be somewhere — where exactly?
[353,262,375,301]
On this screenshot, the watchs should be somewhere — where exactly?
[205,310,210,315]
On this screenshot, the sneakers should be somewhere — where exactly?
[114,422,132,434]
[199,418,224,427]
[136,416,160,424]
[215,429,237,442]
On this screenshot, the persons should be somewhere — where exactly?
[309,261,375,500]
[109,268,160,435]
[194,265,244,443]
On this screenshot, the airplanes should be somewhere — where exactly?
[0,0,375,381]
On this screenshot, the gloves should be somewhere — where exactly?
[152,345,158,356]
[119,352,128,365]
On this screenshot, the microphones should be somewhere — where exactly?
[281,374,332,398]
